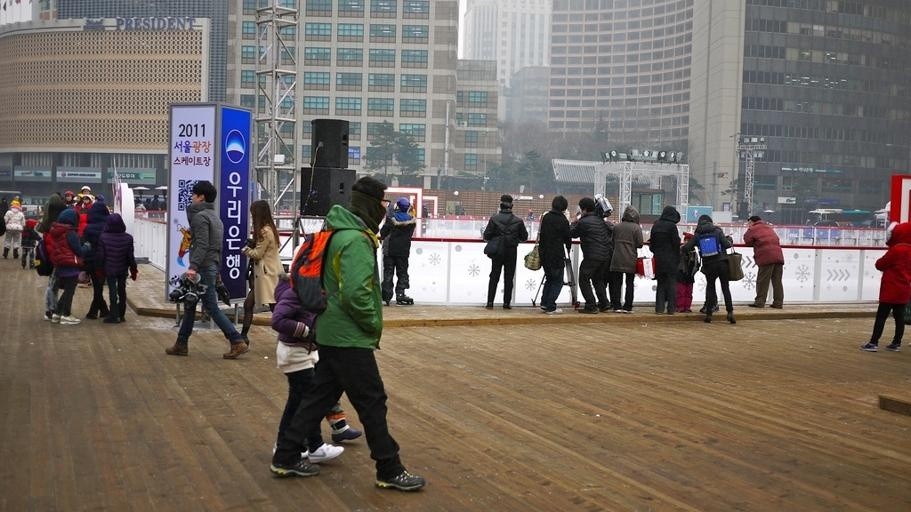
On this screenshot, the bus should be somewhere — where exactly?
[807,208,877,228]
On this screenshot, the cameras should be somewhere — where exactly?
[168,275,200,302]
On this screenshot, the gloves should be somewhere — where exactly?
[131,269,138,280]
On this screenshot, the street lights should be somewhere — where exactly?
[739,135,769,220]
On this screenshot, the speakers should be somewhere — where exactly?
[301,167,356,216]
[311,119,349,167]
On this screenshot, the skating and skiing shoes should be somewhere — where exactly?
[382,293,392,305]
[397,291,413,304]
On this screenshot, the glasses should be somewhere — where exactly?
[380,198,391,208]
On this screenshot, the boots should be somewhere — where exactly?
[13,248,18,256]
[3,247,9,256]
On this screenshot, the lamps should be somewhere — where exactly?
[738,134,774,160]
[599,146,689,164]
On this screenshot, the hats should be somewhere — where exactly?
[64,191,73,197]
[82,186,90,191]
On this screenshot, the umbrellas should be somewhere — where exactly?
[802,206,889,220]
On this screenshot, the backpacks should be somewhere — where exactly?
[291,231,338,315]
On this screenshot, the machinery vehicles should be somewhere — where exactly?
[874,201,891,230]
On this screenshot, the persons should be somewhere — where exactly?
[537,194,736,324]
[327,403,360,442]
[859,221,910,352]
[378,196,415,305]
[269,269,346,464]
[0,184,166,326]
[482,194,528,309]
[163,178,249,359]
[240,199,283,344]
[741,214,783,308]
[269,175,425,494]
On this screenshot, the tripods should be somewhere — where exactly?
[532,243,578,307]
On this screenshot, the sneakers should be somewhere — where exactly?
[43,311,82,326]
[702,303,719,312]
[86,311,125,323]
[165,345,188,356]
[728,313,735,323]
[271,425,362,477]
[860,343,877,352]
[376,470,425,491]
[223,344,248,359]
[885,344,900,352]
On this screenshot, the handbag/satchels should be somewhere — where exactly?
[726,253,743,281]
[33,241,52,276]
[524,246,541,270]
[636,257,655,277]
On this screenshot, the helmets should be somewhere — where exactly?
[397,197,410,208]
